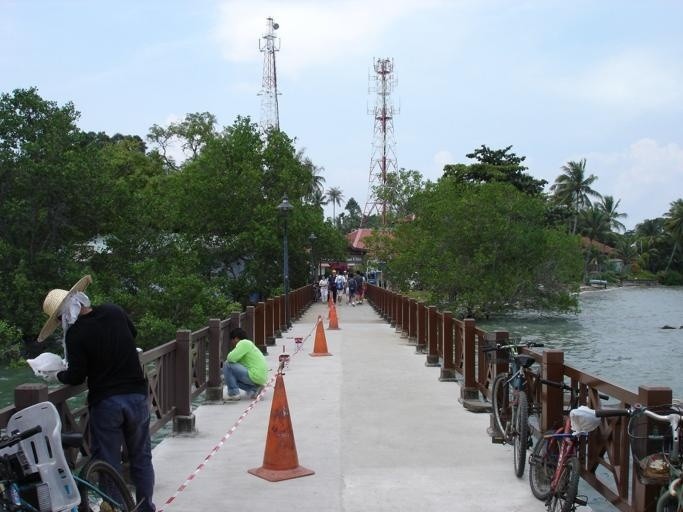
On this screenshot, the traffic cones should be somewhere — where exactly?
[308,315,332,357]
[326,297,340,331]
[247,370,315,483]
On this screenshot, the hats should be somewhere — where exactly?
[37,275,92,343]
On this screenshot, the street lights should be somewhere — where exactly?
[275,189,297,332]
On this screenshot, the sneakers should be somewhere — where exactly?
[223,396,241,402]
[251,386,265,399]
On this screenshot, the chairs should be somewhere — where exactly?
[6,403,81,512]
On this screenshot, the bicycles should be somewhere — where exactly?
[482,339,543,477]
[627,399,682,512]
[0,400,142,512]
[530,374,611,510]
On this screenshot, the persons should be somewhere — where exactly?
[342,271,348,294]
[361,273,368,299]
[37,275,156,511]
[334,271,347,303]
[353,270,363,304]
[348,273,357,307]
[222,327,268,400]
[319,275,329,304]
[327,269,337,304]
[311,276,321,303]
